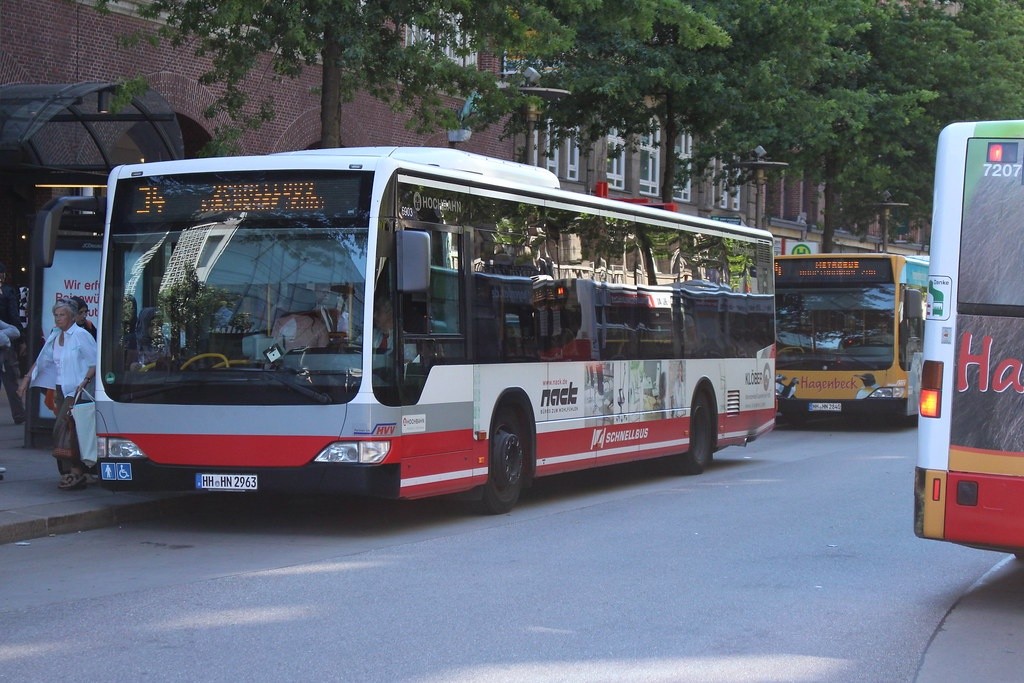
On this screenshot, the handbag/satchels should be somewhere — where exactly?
[73,388,98,468]
[51,385,83,466]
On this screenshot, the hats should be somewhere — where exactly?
[142,308,163,321]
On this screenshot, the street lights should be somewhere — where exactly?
[721,145,789,231]
[497,66,572,168]
[863,189,910,253]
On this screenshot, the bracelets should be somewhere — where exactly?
[84,377,91,383]
[24,375,31,377]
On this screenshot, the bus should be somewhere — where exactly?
[911,117,1024,560]
[34,143,782,517]
[773,249,930,427]
[429,263,772,365]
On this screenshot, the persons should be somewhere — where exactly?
[355,304,417,360]
[272,289,328,350]
[123,295,138,342]
[44,296,97,414]
[0,266,27,424]
[16,299,97,488]
[869,320,894,345]
[125,307,173,371]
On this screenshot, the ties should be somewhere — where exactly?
[380,333,389,352]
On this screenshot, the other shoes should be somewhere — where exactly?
[58,472,97,488]
[0,467,6,472]
[13,411,26,424]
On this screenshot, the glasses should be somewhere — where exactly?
[77,310,90,315]
